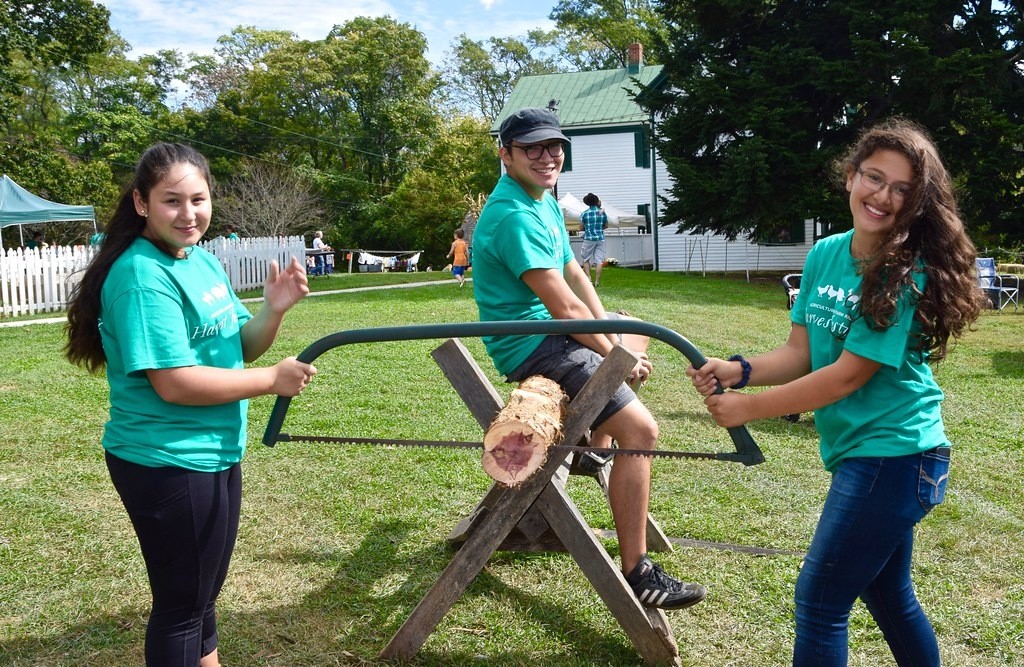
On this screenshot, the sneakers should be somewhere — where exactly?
[574,435,621,477]
[628,555,709,610]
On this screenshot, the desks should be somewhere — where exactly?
[305,251,336,279]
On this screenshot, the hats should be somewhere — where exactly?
[499,106,571,145]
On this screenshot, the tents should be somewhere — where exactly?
[558,192,647,234]
[0,174,98,250]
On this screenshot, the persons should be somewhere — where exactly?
[306,231,334,274]
[579,193,609,287]
[687,128,991,666]
[427,264,433,272]
[26,232,58,275]
[228,224,240,244]
[67,144,317,667]
[473,109,707,610]
[447,229,469,287]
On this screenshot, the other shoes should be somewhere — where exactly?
[460,278,466,288]
[594,280,600,287]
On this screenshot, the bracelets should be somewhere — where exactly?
[730,354,751,391]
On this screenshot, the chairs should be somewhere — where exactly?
[975,258,1019,315]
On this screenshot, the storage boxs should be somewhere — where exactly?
[368,265,380,271]
[358,264,367,272]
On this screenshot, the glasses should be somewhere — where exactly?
[855,164,911,202]
[511,140,565,163]
